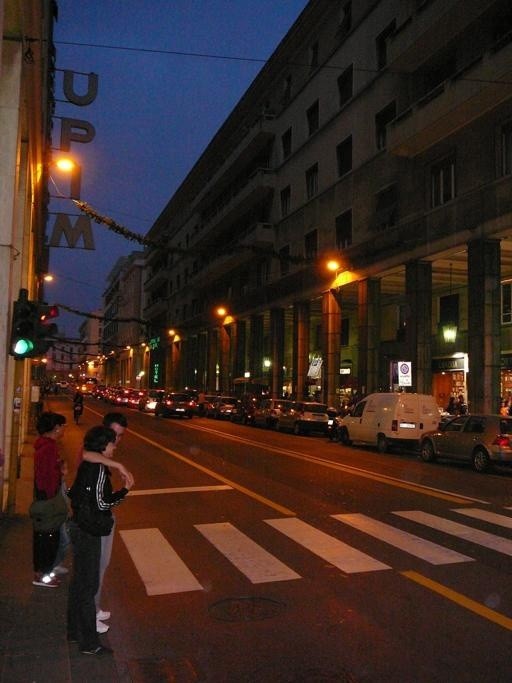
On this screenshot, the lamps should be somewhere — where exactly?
[442,263,457,343]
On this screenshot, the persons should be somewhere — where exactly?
[72,390,84,419]
[52,413,73,575]
[446,397,458,415]
[31,411,63,588]
[457,395,467,415]
[82,412,131,634]
[198,390,206,418]
[66,426,135,658]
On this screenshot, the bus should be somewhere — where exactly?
[59,379,67,388]
[76,376,97,396]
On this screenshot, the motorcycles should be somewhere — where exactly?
[327,404,345,441]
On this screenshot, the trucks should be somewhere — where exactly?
[335,391,441,449]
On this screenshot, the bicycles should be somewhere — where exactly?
[73,400,83,424]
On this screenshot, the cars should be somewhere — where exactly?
[91,384,167,412]
[156,391,196,419]
[275,401,332,436]
[194,392,293,431]
[417,413,512,473]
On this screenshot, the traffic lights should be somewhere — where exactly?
[31,301,59,357]
[9,300,38,359]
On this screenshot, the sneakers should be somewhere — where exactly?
[33,565,68,589]
[97,622,109,634]
[81,646,112,657]
[95,610,111,620]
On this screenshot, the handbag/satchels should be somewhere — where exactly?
[29,496,69,533]
[74,488,114,536]
